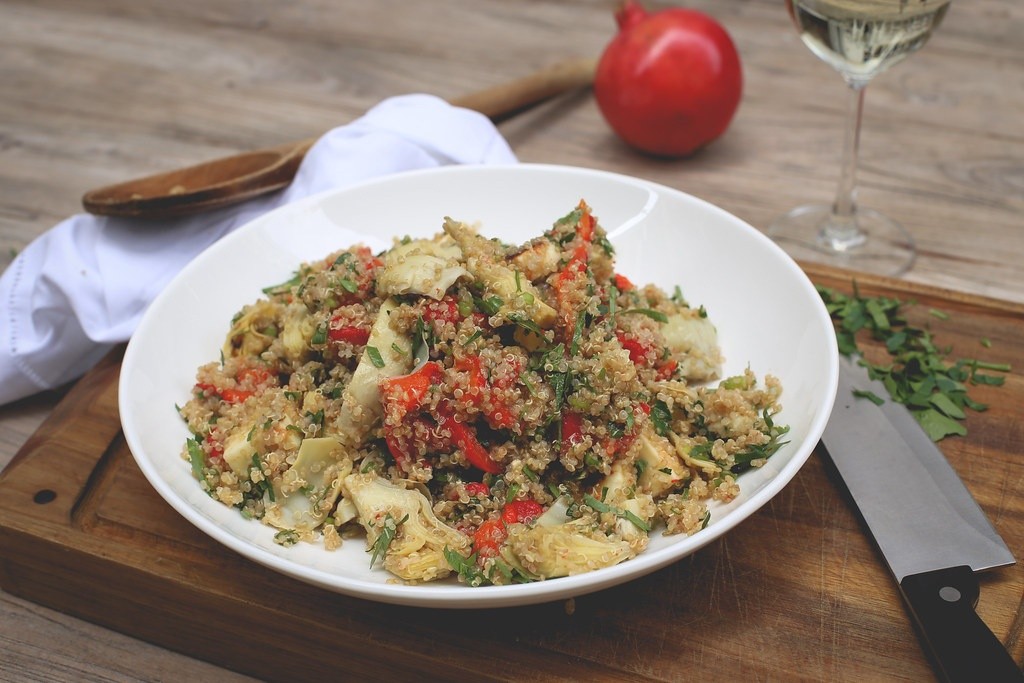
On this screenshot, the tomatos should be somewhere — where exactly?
[594,9,742,156]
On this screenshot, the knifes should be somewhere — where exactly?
[821,352,1024,683]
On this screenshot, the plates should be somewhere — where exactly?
[117,162,840,609]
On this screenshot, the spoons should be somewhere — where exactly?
[81,46,598,220]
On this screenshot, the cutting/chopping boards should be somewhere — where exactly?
[0,260,1024,683]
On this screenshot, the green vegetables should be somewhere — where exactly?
[814,279,1011,440]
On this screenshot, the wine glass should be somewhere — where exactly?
[763,0,954,284]
